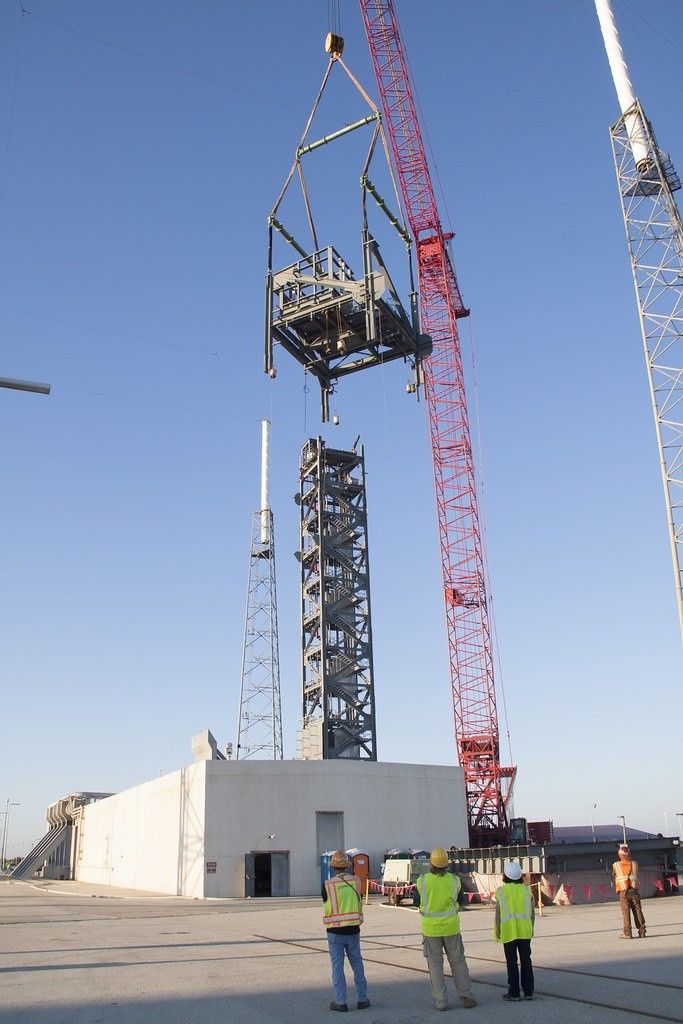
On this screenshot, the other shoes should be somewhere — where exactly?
[463,997,477,1008]
[502,994,521,1001]
[357,998,370,1009]
[639,932,645,938]
[330,1001,348,1011]
[525,995,533,1000]
[618,934,633,939]
[436,1006,446,1010]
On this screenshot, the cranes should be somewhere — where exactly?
[354,0,555,847]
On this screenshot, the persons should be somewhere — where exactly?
[611,848,648,940]
[321,850,372,1013]
[494,862,536,1001]
[413,848,478,1011]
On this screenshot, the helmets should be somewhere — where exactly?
[431,848,449,868]
[504,862,523,880]
[618,846,631,859]
[330,851,351,868]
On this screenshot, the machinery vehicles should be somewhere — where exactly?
[380,858,450,905]
